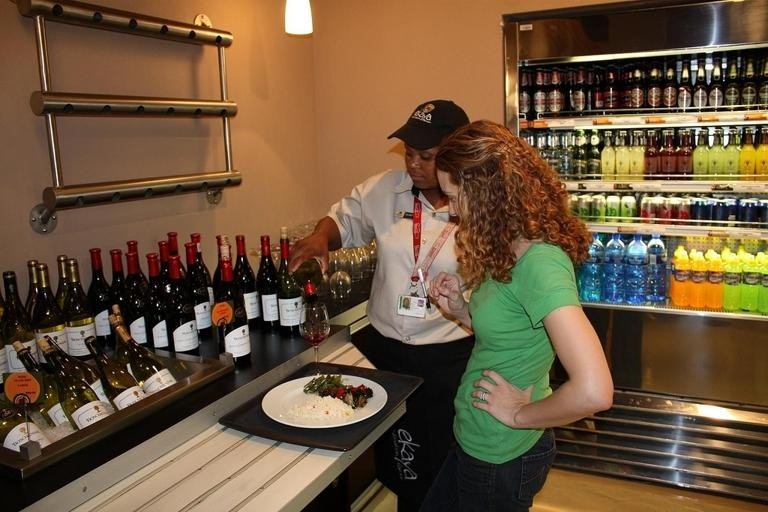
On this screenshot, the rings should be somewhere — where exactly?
[482,391,486,401]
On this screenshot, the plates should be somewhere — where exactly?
[262,374,389,431]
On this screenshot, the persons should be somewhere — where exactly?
[400,298,411,309]
[285,100,471,512]
[418,119,615,512]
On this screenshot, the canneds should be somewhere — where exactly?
[563,192,768,229]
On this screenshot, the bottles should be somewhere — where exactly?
[0,255,177,457]
[518,48,768,116]
[87,226,306,369]
[518,125,767,182]
[569,232,768,320]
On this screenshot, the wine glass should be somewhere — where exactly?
[248,218,377,300]
[298,302,331,375]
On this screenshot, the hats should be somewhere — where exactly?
[387,98,472,149]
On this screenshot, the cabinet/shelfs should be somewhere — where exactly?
[502,0,768,411]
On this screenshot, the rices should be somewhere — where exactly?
[278,393,353,424]
